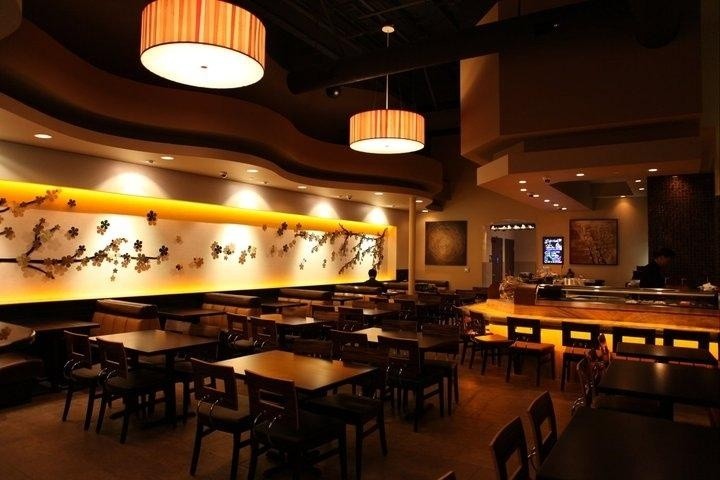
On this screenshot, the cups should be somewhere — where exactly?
[544,267,551,276]
[564,277,586,285]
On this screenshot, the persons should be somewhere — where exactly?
[638,246,676,288]
[362,269,387,292]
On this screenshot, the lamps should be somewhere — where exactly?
[138,0,266,89]
[347,23,429,155]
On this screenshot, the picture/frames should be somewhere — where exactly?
[569,220,618,265]
[543,237,564,264]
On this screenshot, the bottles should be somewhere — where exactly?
[567,268,574,278]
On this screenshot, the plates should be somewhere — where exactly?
[644,287,674,291]
